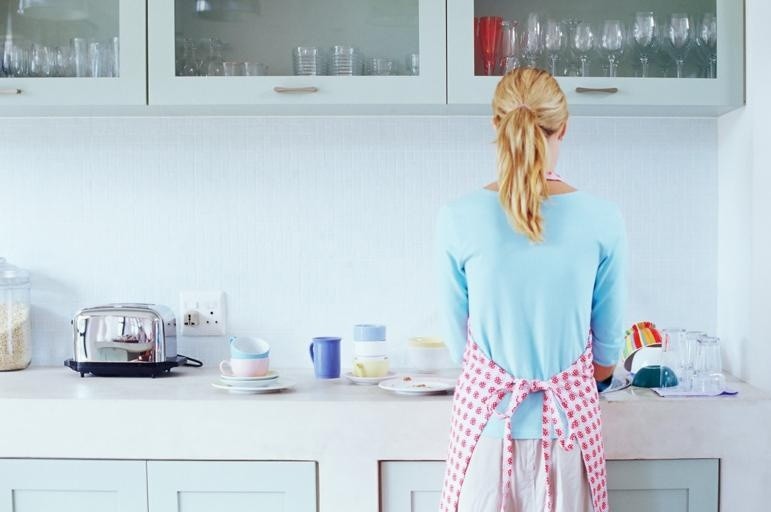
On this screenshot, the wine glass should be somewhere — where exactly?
[475,12,716,78]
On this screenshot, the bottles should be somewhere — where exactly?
[0,257,31,372]
[404,334,449,376]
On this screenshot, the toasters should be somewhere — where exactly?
[64,301,187,378]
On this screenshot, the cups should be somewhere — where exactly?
[175,35,418,77]
[352,323,390,378]
[309,337,343,380]
[0,35,120,77]
[660,327,726,397]
[219,335,270,376]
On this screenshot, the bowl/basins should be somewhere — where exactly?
[622,320,678,388]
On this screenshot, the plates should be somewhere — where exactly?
[343,371,398,385]
[211,371,296,394]
[378,375,456,396]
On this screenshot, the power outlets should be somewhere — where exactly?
[163,292,226,339]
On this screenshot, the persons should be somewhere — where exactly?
[438,65,624,512]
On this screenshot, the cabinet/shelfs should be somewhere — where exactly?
[0,366,770,511]
[0,0,746,116]
